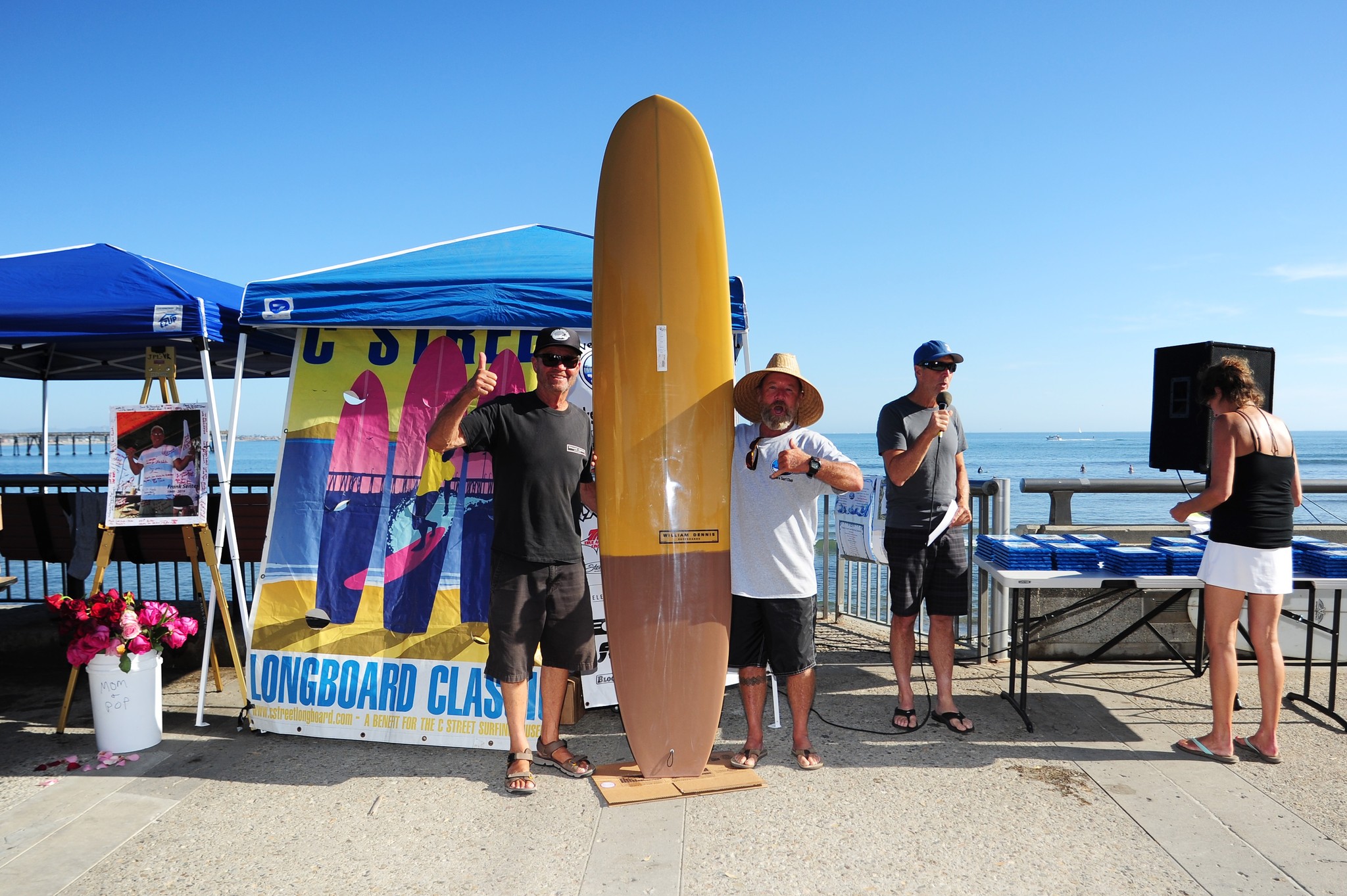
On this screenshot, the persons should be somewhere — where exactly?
[124,426,195,518]
[425,327,601,795]
[590,353,863,769]
[876,341,977,733]
[1169,353,1301,763]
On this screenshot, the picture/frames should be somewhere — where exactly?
[105,402,210,527]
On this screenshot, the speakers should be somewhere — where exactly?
[1148,341,1276,475]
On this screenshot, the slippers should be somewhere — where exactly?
[931,709,976,733]
[1232,735,1284,764]
[1175,736,1240,762]
[892,706,919,732]
[789,746,825,770]
[730,747,768,769]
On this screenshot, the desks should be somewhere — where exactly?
[967,552,1347,734]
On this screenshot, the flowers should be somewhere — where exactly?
[44,587,200,674]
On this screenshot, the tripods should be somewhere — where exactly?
[1077,475,1255,677]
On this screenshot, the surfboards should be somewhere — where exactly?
[592,93,735,779]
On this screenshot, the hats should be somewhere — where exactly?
[732,352,824,429]
[533,326,583,356]
[913,340,964,366]
[151,425,164,435]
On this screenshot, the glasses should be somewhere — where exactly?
[745,436,769,471]
[917,361,957,372]
[151,432,164,436]
[533,354,580,368]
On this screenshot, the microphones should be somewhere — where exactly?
[936,392,952,443]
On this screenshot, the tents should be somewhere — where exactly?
[194,225,779,731]
[0,244,299,729]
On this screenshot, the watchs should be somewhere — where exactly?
[807,456,822,479]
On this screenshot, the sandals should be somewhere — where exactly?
[504,747,537,795]
[532,735,597,778]
